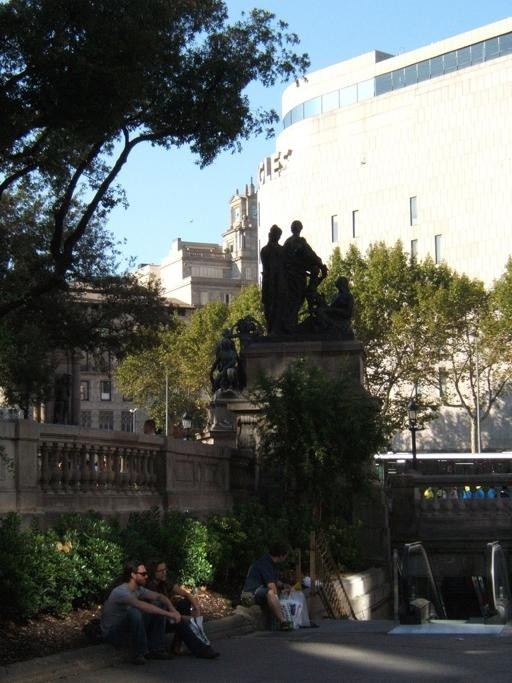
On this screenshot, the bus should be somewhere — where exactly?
[375,445,512,498]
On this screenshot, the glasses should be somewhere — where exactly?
[135,572,148,576]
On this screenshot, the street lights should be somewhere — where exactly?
[128,406,137,432]
[407,394,422,470]
[179,410,195,440]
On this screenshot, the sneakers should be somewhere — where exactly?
[150,650,175,659]
[135,651,145,664]
[198,646,220,659]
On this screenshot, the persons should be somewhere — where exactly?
[238,540,321,632]
[99,559,181,665]
[146,557,220,658]
[271,220,323,333]
[258,224,285,333]
[207,337,241,393]
[309,276,354,334]
[142,418,159,436]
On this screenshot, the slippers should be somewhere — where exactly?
[280,621,288,630]
[299,621,320,628]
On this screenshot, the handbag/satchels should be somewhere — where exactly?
[179,616,211,656]
[271,585,311,631]
